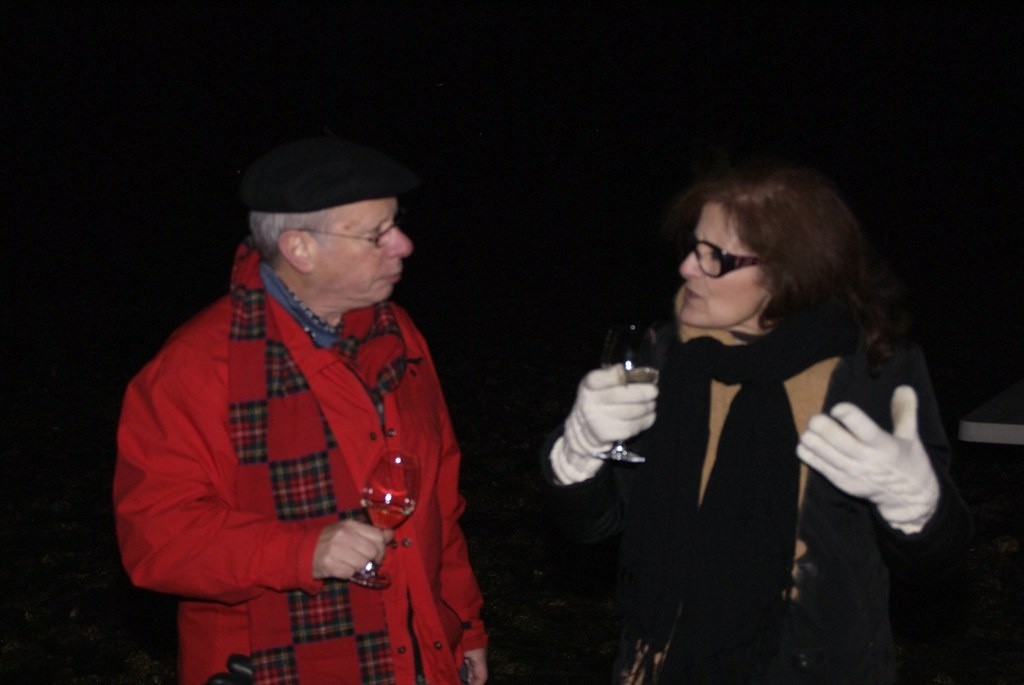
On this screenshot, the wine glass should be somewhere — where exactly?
[349,449,421,589]
[599,325,665,462]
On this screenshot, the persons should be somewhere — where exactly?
[535,156,975,685]
[112,141,491,685]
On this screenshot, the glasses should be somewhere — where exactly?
[282,219,407,249]
[689,232,763,279]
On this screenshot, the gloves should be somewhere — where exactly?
[550,364,659,486]
[796,385,940,535]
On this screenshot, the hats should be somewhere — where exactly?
[233,136,425,214]
